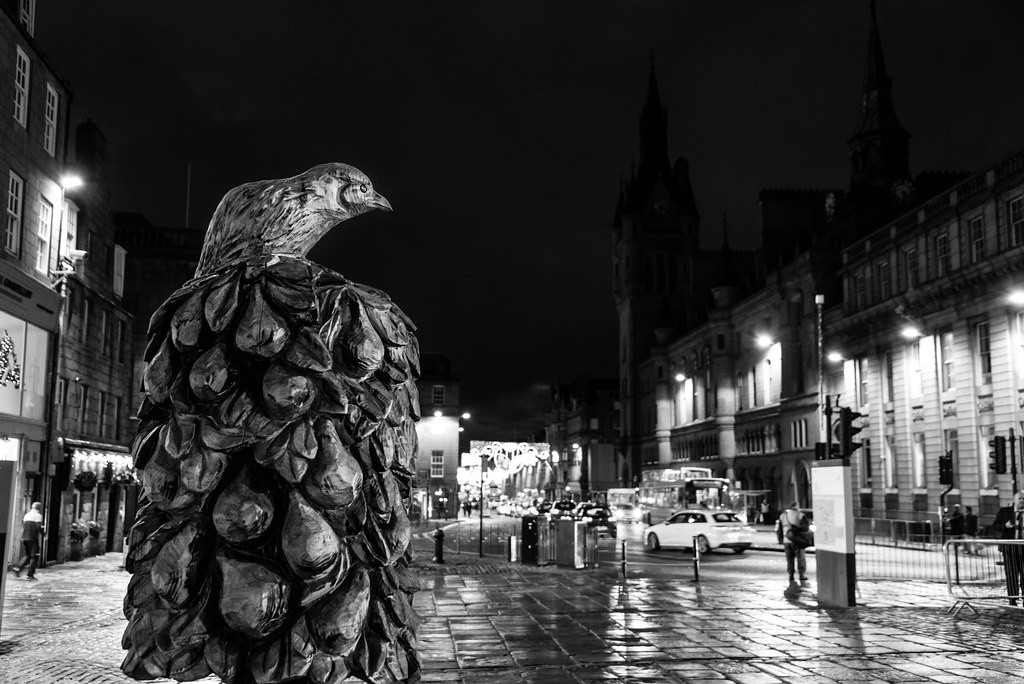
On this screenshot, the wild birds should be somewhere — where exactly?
[192,160,392,280]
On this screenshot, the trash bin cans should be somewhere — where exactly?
[521,514,550,566]
[555,520,588,569]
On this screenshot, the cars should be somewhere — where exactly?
[492,499,616,538]
[609,504,640,524]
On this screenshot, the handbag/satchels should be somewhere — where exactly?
[760,514,763,522]
[975,536,983,550]
[787,529,814,550]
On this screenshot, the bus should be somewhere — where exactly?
[639,478,731,525]
[643,467,713,480]
[607,488,640,507]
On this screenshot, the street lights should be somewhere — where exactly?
[823,408,840,459]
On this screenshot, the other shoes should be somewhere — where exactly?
[790,576,794,580]
[1010,601,1017,605]
[25,576,36,580]
[13,567,19,577]
[800,575,808,580]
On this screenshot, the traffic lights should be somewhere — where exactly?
[988,437,1007,475]
[840,408,863,456]
[938,457,950,483]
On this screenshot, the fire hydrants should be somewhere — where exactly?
[433,529,444,562]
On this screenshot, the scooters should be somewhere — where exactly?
[934,508,952,540]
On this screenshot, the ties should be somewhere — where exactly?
[1018,512,1022,539]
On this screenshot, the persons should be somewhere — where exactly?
[948,503,965,551]
[992,490,1024,607]
[432,496,475,521]
[777,501,811,580]
[962,506,979,554]
[760,499,769,523]
[12,501,44,581]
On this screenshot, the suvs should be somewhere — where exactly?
[643,508,753,555]
